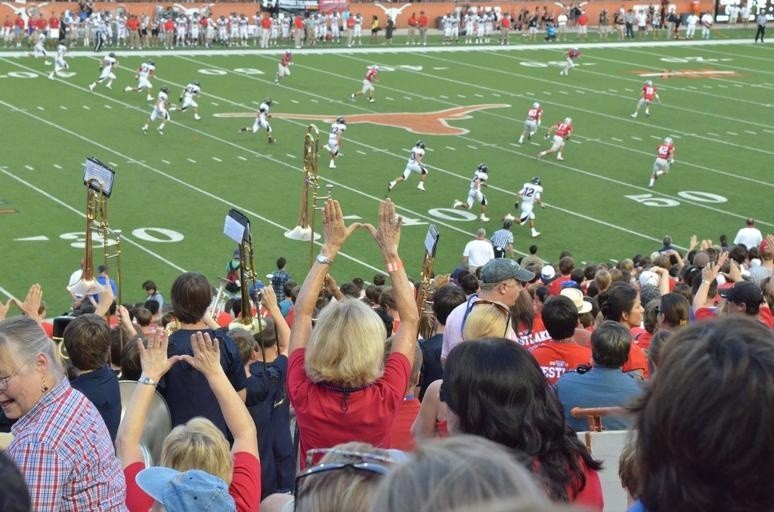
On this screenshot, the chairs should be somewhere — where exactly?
[119,379,171,461]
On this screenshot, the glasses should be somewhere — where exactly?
[0,365,25,390]
[294,462,386,512]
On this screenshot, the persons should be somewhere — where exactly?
[123,60,201,135]
[2,0,768,53]
[387,140,428,192]
[648,136,675,188]
[536,117,573,162]
[30,29,70,78]
[453,164,490,222]
[351,65,378,102]
[499,176,545,237]
[518,102,544,144]
[629,80,661,120]
[238,96,275,143]
[560,47,581,73]
[88,52,118,91]
[2,195,773,512]
[274,49,292,85]
[321,117,347,168]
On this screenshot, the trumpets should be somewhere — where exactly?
[210,276,228,322]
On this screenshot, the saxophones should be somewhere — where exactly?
[415,247,437,341]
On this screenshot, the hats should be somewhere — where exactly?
[561,288,593,314]
[479,259,536,283]
[542,265,555,279]
[135,467,236,512]
[718,282,764,304]
[694,254,709,268]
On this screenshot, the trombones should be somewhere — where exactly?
[229,223,267,363]
[284,122,333,323]
[67,177,124,378]
